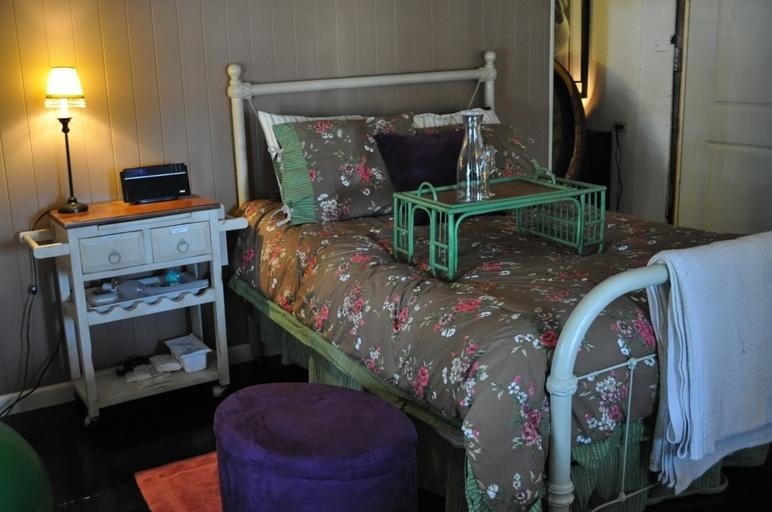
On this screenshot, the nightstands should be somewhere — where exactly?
[17,195,250,430]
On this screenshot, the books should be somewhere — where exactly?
[124,365,176,392]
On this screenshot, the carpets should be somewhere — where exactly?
[133,449,223,511]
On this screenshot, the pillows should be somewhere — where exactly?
[413,120,541,187]
[375,121,477,225]
[256,106,503,152]
[271,111,415,227]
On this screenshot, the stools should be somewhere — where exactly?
[209,383,420,511]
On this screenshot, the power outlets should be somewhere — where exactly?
[613,120,628,134]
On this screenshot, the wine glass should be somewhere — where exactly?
[471,144,497,199]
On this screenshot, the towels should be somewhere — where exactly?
[645,230,771,499]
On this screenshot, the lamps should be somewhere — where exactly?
[40,65,90,214]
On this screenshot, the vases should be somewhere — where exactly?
[452,111,494,199]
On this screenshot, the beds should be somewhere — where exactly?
[218,46,771,511]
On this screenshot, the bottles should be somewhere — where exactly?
[455,112,485,204]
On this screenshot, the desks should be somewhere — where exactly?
[390,169,610,283]
